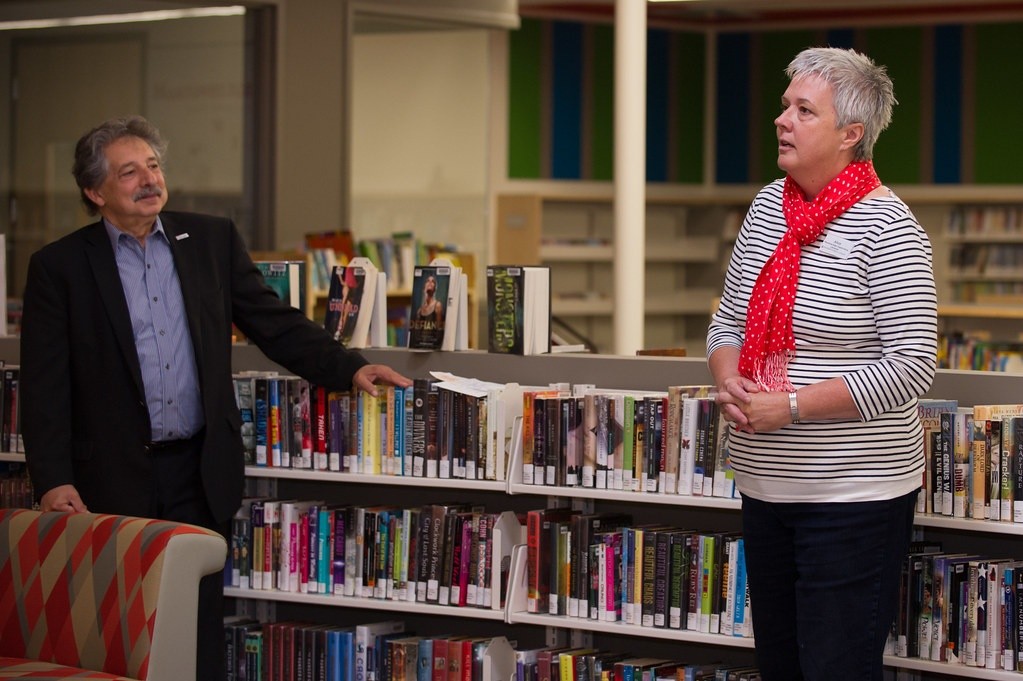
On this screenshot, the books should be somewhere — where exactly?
[0,202,1023,681]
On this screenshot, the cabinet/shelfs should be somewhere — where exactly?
[495,180,1022,372]
[1,334,1022,681]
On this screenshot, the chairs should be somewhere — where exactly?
[0,507,226,681]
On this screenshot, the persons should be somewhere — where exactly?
[412,276,444,348]
[18,115,413,681]
[706,47,938,681]
[335,265,363,340]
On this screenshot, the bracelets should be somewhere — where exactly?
[788,392,800,423]
